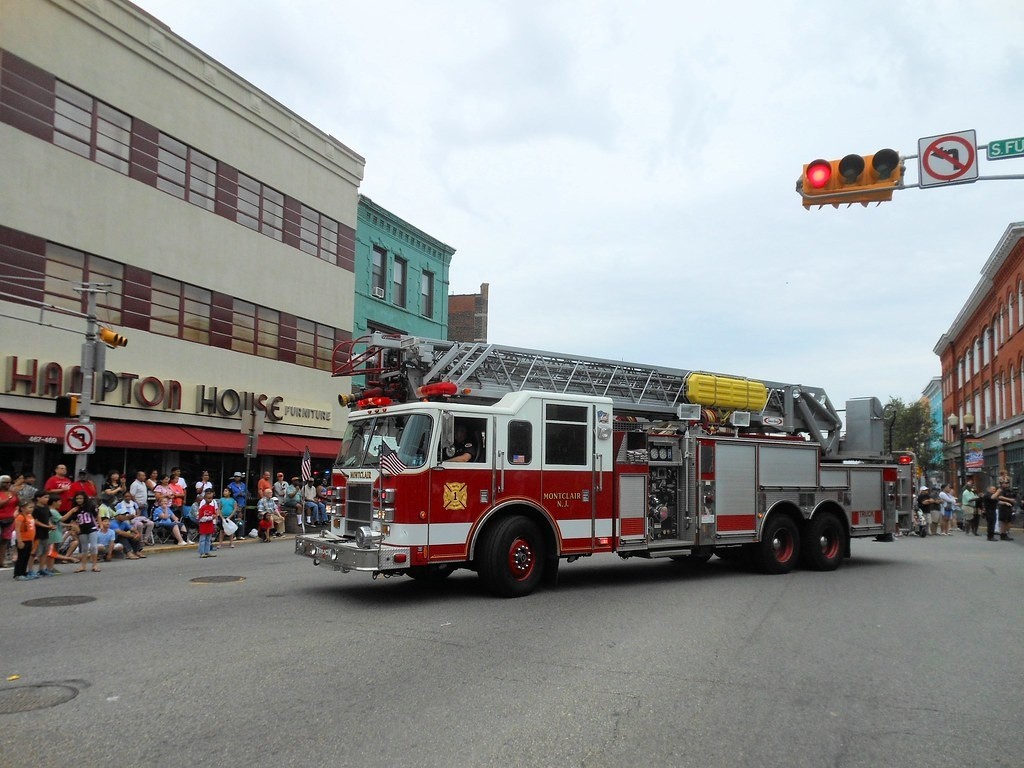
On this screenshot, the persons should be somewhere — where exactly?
[443,425,477,462]
[0,464,331,581]
[893,474,1015,542]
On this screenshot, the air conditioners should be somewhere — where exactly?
[372,287,385,298]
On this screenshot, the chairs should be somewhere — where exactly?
[465,426,484,463]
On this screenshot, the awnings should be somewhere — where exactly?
[0,411,372,459]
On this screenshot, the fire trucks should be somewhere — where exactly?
[294,329,912,600]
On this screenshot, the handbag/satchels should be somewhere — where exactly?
[222,518,238,535]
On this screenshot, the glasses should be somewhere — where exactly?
[2,481,12,484]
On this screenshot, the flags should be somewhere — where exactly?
[301,447,311,481]
[380,440,407,476]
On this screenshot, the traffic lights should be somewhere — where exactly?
[802,149,902,197]
[55,397,77,417]
[98,327,127,348]
[800,191,895,211]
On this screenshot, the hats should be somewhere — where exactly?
[78,468,88,474]
[920,486,929,491]
[308,478,314,481]
[115,510,130,517]
[292,477,299,481]
[234,472,242,478]
[273,497,279,501]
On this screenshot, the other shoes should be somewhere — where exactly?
[264,538,271,543]
[200,554,207,558]
[276,533,285,538]
[230,544,234,547]
[895,526,1013,542]
[217,544,222,548]
[206,552,216,557]
[144,540,154,546]
[2,549,146,580]
[296,518,332,529]
[176,533,245,545]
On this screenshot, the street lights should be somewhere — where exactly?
[948,412,974,502]
